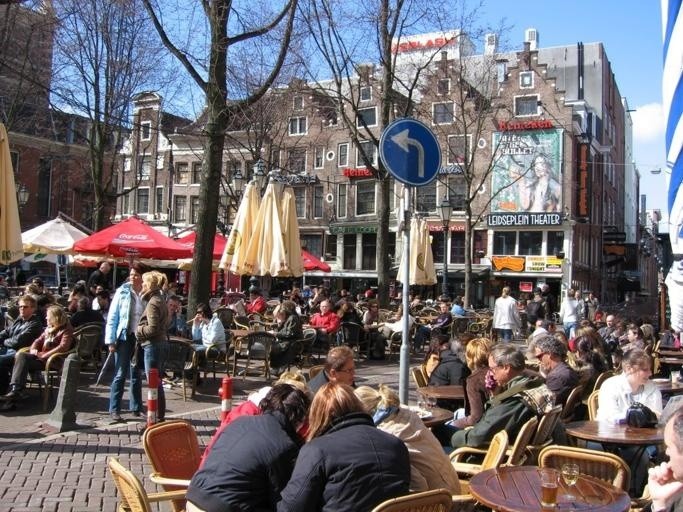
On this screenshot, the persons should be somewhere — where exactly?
[629,407,683,511]
[276,381,410,511]
[0,265,118,412]
[388,279,609,430]
[201,373,311,466]
[512,152,561,214]
[104,263,147,422]
[607,313,682,371]
[353,383,461,497]
[586,349,662,493]
[167,279,384,387]
[438,343,553,478]
[137,270,168,420]
[185,383,310,511]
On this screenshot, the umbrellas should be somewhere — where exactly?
[219,179,263,275]
[396,215,438,286]
[175,226,230,259]
[0,116,24,268]
[243,170,286,280]
[279,182,304,280]
[16,217,90,284]
[75,217,191,265]
[300,248,331,271]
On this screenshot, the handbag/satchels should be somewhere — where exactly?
[627,403,658,426]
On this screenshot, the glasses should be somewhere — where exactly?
[338,366,356,373]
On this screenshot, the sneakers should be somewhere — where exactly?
[0,384,32,413]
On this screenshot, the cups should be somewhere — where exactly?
[540,469,560,507]
[235,288,238,292]
[671,371,680,383]
[288,290,291,294]
[228,288,232,293]
[283,291,286,296]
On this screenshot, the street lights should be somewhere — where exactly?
[630,154,662,235]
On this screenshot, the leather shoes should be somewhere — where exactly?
[110,411,121,420]
[133,411,149,418]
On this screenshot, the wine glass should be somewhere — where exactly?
[418,400,436,418]
[562,464,579,501]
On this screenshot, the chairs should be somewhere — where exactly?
[15,332,83,410]
[71,324,102,373]
